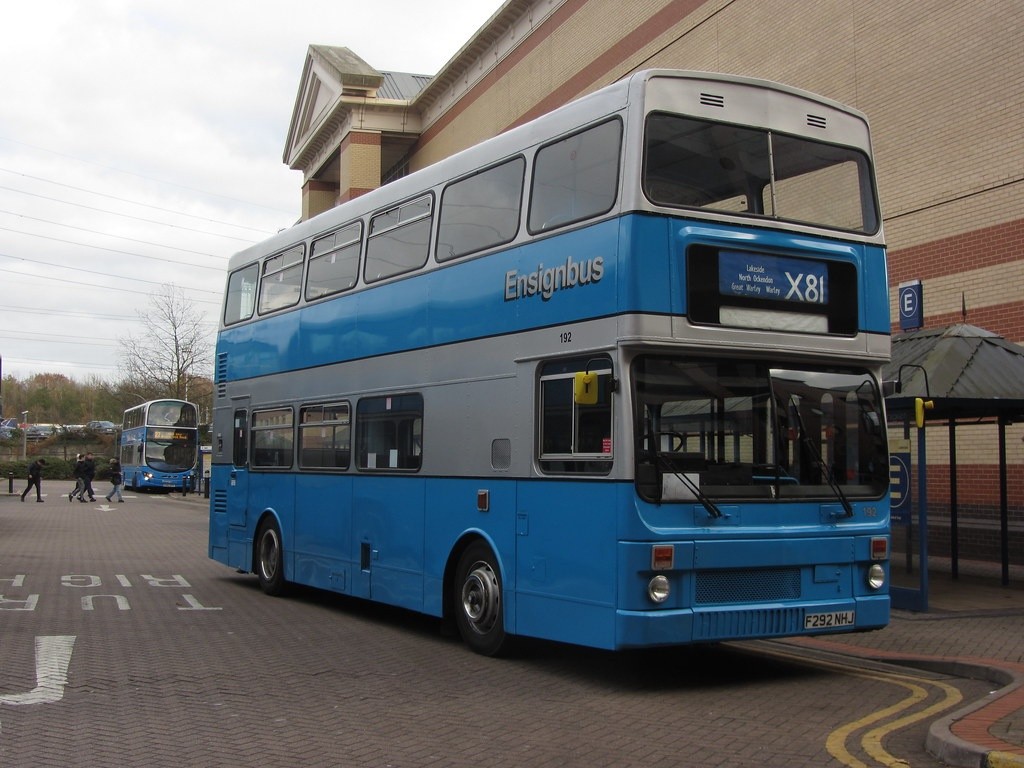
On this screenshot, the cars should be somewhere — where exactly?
[1,418,117,438]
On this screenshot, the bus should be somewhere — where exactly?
[207,70,938,659]
[118,398,205,492]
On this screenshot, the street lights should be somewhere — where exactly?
[181,348,193,402]
[111,392,148,403]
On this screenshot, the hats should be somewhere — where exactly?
[110,458,117,463]
[39,459,45,465]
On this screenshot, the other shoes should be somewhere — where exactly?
[80,498,88,502]
[77,496,81,501]
[69,494,73,502]
[90,498,96,501]
[21,494,25,502]
[106,497,112,502]
[119,500,124,502]
[37,497,44,502]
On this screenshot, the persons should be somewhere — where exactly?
[105,459,124,502]
[20,459,45,502]
[69,452,96,503]
